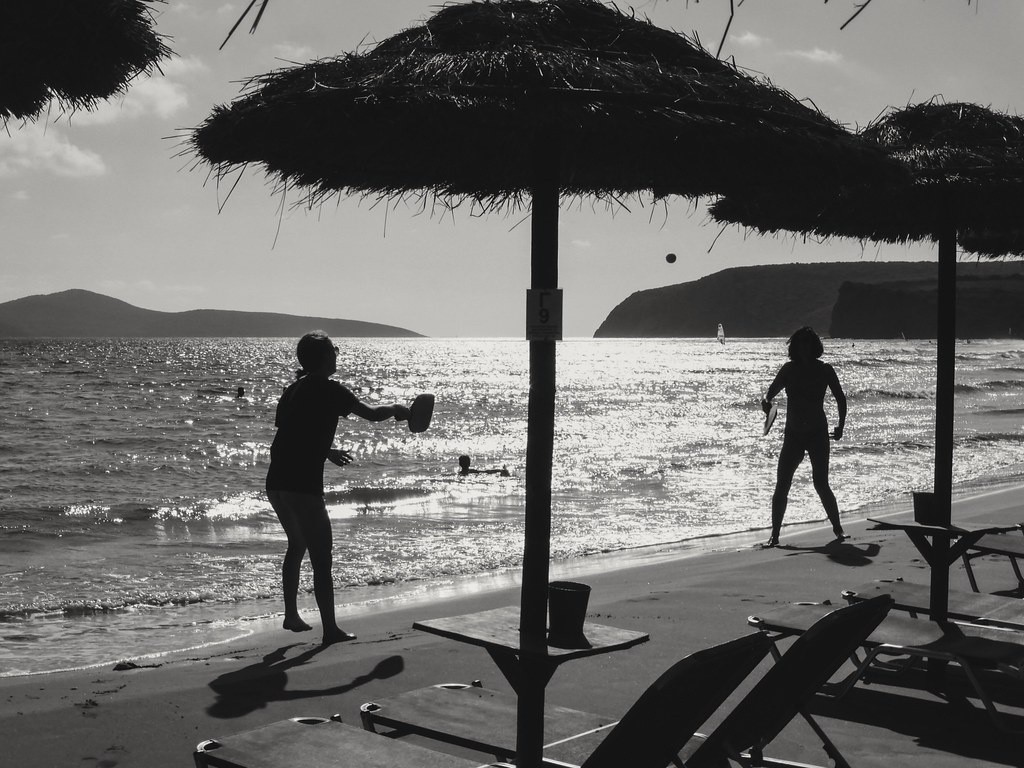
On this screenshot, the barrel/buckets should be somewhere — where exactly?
[548,579,591,641]
[912,490,933,523]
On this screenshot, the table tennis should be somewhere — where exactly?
[666,253,677,263]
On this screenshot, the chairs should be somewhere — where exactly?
[951,533,1024,597]
[743,601,1024,731]
[359,595,894,768]
[193,633,774,767]
[842,577,1024,632]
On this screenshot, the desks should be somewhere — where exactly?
[865,515,1020,617]
[413,605,650,768]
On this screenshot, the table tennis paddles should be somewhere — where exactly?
[764,402,777,436]
[394,394,435,433]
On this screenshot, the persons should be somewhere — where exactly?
[929,341,931,343]
[457,454,504,477]
[266,330,410,643]
[967,338,971,343]
[761,328,852,548]
[852,343,855,347]
[234,386,245,400]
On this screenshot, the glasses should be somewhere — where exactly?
[328,346,340,358]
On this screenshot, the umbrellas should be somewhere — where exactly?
[705,106,1024,602]
[0,0,164,116]
[191,0,854,641]
